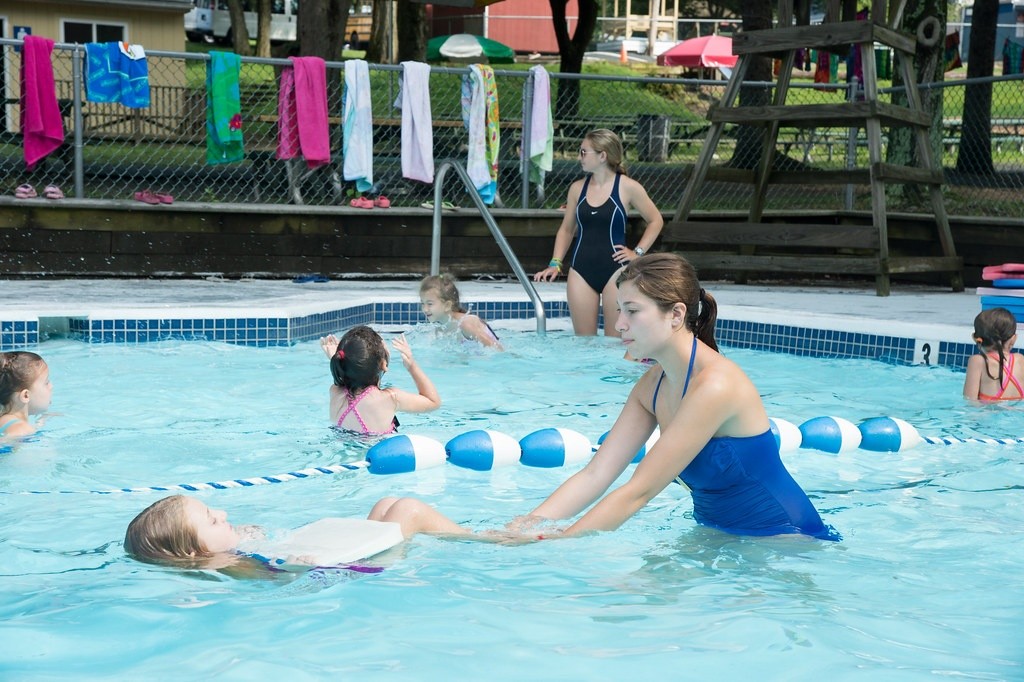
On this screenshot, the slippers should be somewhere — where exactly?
[350,196,390,209]
[421,200,461,210]
[472,273,536,283]
[134,189,173,204]
[206,273,230,281]
[292,273,329,283]
[239,273,256,282]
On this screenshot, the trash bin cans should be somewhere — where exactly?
[637,114,669,162]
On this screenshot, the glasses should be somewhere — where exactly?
[578,145,602,157]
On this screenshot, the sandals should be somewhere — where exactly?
[15,183,37,197]
[44,183,64,198]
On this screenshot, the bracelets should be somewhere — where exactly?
[548,258,563,272]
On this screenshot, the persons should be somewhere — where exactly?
[0,350,51,436]
[531,252,826,536]
[123,494,452,558]
[963,307,1024,402]
[420,273,501,350]
[534,129,663,336]
[320,326,441,434]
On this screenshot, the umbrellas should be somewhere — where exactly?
[425,33,515,59]
[657,35,739,67]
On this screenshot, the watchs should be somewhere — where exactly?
[634,246,644,257]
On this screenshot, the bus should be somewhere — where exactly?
[184,0,297,46]
